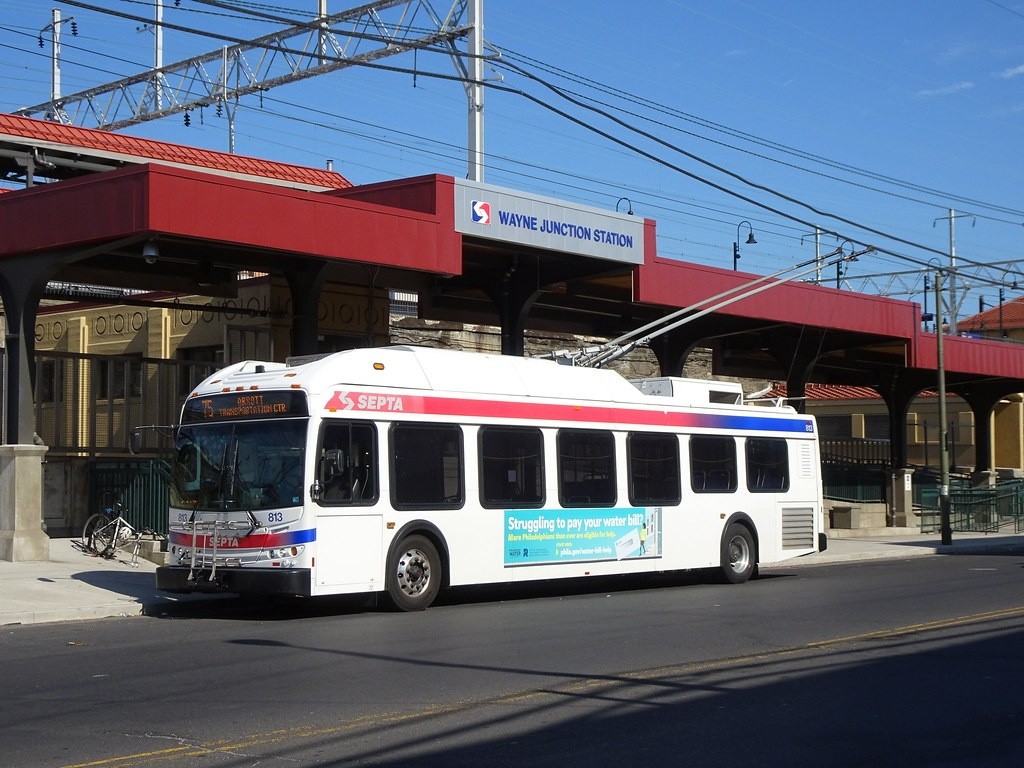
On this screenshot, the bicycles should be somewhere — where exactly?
[79,498,168,568]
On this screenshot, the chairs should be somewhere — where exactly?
[581,472,612,503]
[692,469,732,489]
[749,465,785,488]
[344,463,369,500]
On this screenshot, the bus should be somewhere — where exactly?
[129,343,833,613]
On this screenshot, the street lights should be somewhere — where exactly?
[836,239,859,290]
[997,271,1021,340]
[922,257,947,332]
[731,220,758,272]
